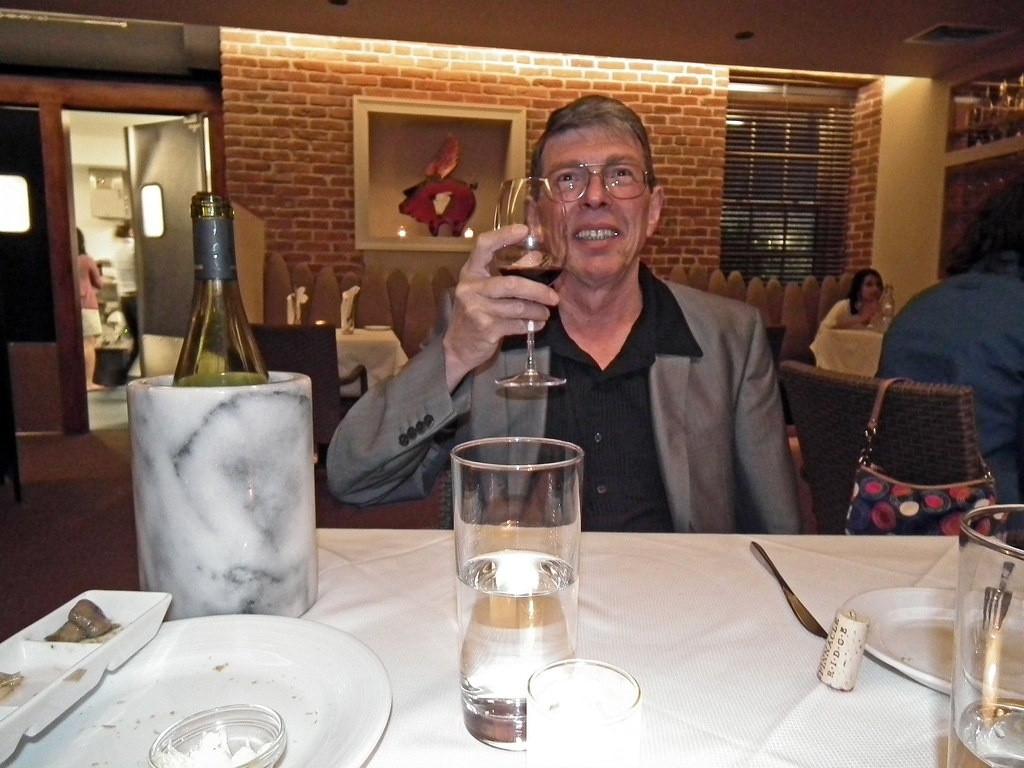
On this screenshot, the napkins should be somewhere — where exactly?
[286,285,309,322]
[341,285,360,322]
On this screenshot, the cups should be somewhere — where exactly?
[526,658,643,767]
[125,373,319,621]
[449,436,583,753]
[950,503,1024,767]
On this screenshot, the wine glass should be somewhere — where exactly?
[490,177,567,387]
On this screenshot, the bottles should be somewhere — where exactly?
[966,74,1024,148]
[172,191,270,387]
[874,283,895,332]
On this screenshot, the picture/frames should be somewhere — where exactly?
[352,94,528,250]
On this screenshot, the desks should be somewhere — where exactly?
[331,325,408,401]
[808,328,885,377]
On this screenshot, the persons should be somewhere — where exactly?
[820,268,885,332]
[77,229,104,391]
[325,94,804,535]
[107,221,142,386]
[876,182,1024,531]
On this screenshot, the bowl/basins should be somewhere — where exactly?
[146,704,287,767]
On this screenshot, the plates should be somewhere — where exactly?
[0,589,172,765]
[0,614,393,768]
[842,587,1023,702]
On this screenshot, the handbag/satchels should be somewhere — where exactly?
[846,377,996,536]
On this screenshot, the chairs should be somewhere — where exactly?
[222,322,367,445]
[778,359,985,536]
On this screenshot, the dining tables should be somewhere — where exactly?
[298,523,1024,768]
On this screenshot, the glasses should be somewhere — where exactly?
[540,162,653,200]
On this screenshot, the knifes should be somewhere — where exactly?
[748,541,828,638]
